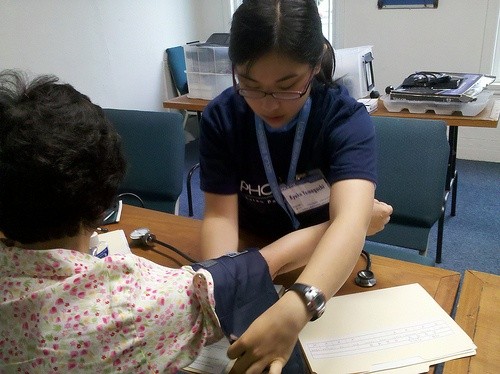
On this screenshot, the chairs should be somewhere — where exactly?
[356,116,450,266]
[164,45,190,96]
[102,109,186,217]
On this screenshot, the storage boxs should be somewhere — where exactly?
[182,44,239,101]
[331,44,376,99]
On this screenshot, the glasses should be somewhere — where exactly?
[232,43,327,100]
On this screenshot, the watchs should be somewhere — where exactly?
[285,283,326,321]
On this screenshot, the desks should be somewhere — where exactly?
[163,92,500,262]
[90,205,462,374]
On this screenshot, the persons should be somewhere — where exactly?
[0,70,393,374]
[198,0,377,374]
